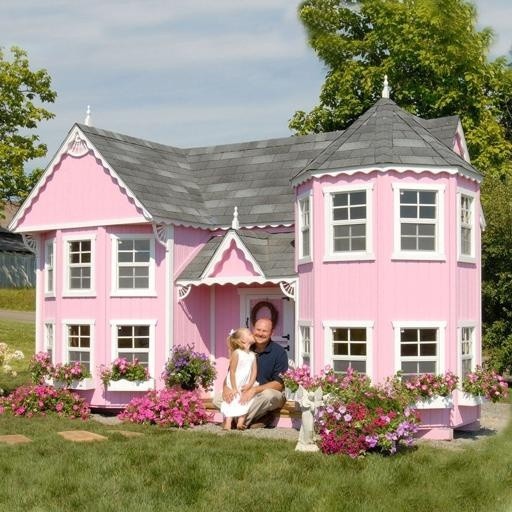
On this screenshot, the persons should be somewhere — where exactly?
[219,327,259,432]
[213,318,288,429]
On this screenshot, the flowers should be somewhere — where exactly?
[100,359,150,381]
[394,372,459,399]
[29,352,93,381]
[278,365,364,400]
[462,365,509,404]
[162,344,214,392]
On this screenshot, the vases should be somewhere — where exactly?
[40,375,94,391]
[105,383,155,391]
[403,394,454,409]
[460,390,489,406]
